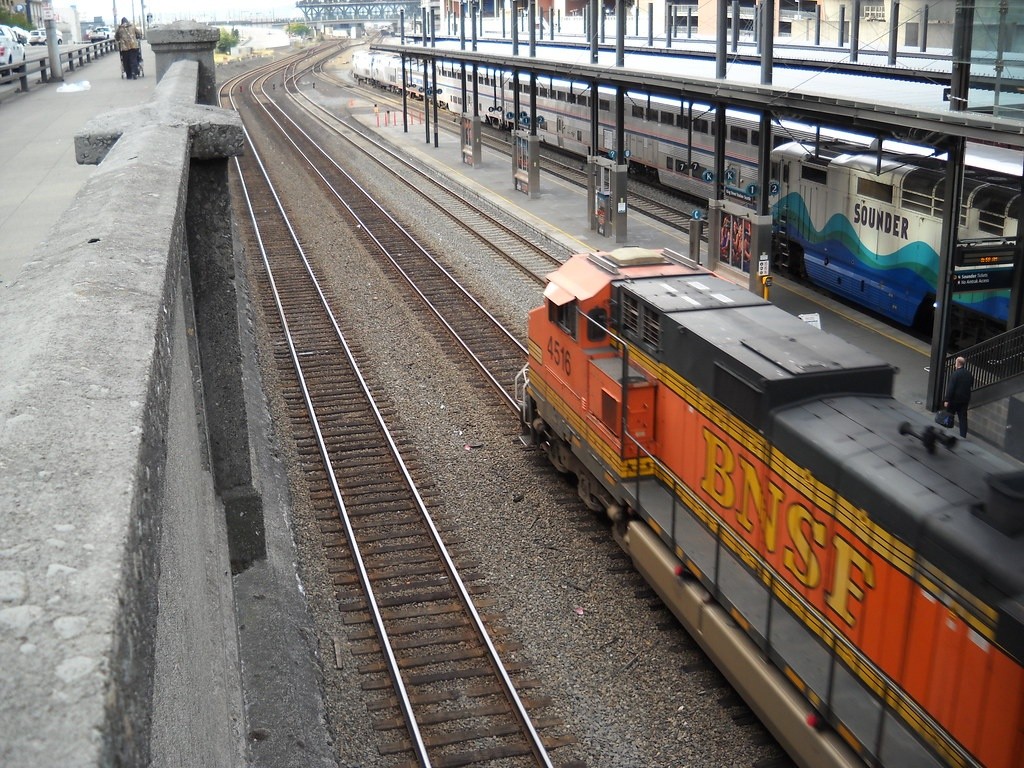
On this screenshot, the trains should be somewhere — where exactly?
[769,138,1023,329]
[516,245,1023,768]
[350,49,1024,209]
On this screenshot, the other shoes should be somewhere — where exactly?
[132,72,137,79]
[126,74,132,79]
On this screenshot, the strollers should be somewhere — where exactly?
[118,38,144,80]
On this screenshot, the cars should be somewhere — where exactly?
[0,24,26,83]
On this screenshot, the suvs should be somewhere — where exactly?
[30,28,63,46]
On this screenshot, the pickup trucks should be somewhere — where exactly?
[89,27,116,43]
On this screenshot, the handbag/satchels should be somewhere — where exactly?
[935,410,954,428]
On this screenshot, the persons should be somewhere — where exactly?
[720,216,751,275]
[114,17,141,80]
[944,357,973,439]
